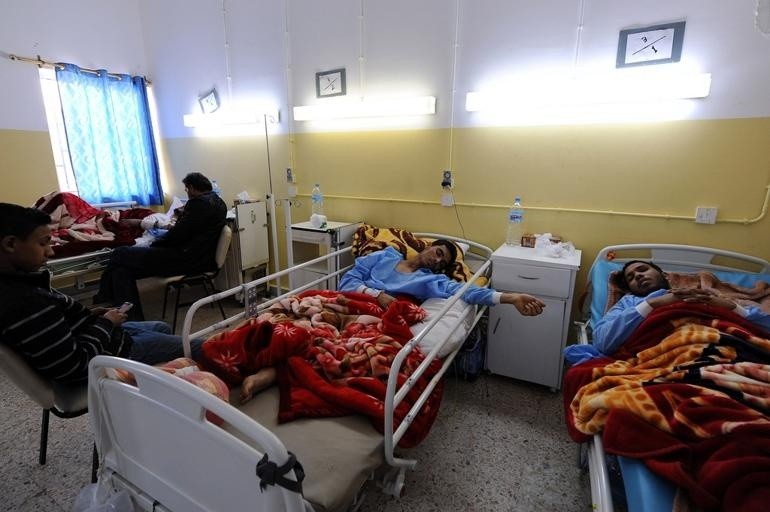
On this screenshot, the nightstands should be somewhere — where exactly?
[214,223,242,301]
[483,259,583,394]
[285,229,340,297]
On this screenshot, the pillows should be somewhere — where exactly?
[163,197,188,217]
[454,241,470,253]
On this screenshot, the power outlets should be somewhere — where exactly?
[441,171,455,191]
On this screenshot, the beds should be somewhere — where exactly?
[84,229,496,511]
[51,246,112,292]
[572,241,769,510]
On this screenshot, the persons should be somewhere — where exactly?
[1,202,208,392]
[92,172,227,321]
[121,203,186,230]
[591,258,770,357]
[239,238,548,407]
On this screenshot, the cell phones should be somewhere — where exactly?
[120,301,133,314]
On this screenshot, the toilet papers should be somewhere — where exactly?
[310,214,329,230]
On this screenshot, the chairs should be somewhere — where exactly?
[158,225,230,334]
[0,343,100,485]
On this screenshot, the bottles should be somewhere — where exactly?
[505,197,524,247]
[311,183,325,216]
[212,180,219,195]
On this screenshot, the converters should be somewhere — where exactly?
[442,182,450,186]
